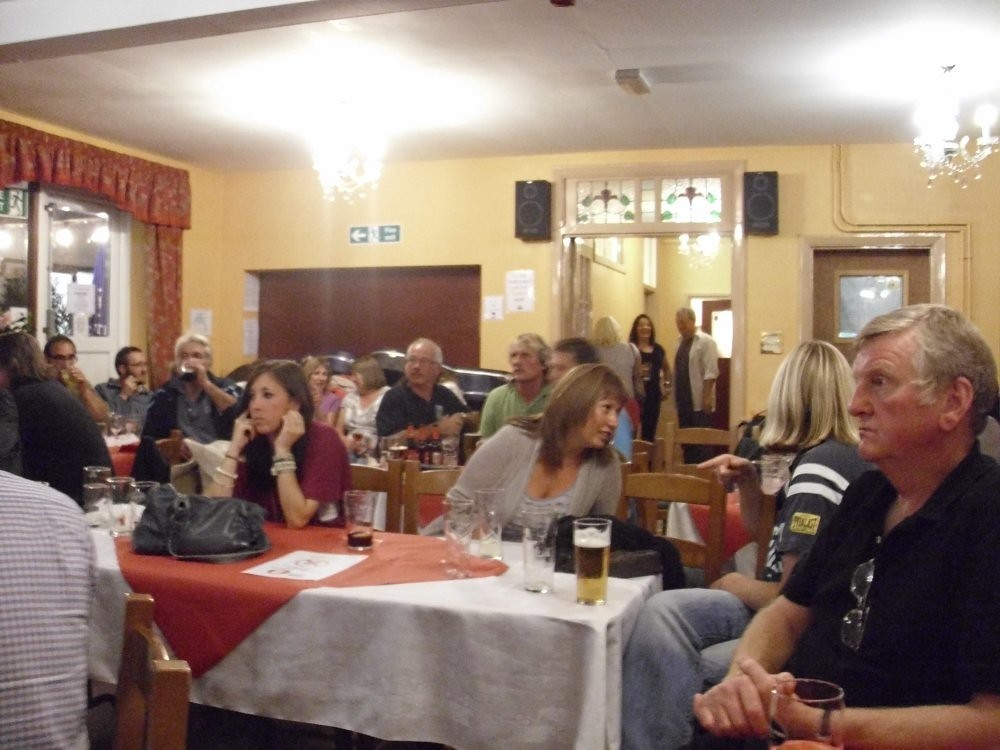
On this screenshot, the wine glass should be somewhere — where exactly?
[109,415,124,440]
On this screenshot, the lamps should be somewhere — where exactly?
[912,98,1000,191]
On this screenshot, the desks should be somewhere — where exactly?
[92,522,663,750]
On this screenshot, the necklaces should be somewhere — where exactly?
[540,465,569,487]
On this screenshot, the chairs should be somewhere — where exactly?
[87,420,777,750]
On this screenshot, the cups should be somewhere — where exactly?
[734,484,741,506]
[522,509,557,593]
[124,420,137,444]
[573,518,612,605]
[106,476,135,537]
[353,431,362,450]
[643,362,650,380]
[477,487,505,560]
[61,368,81,391]
[442,499,474,578]
[769,679,847,750]
[96,422,107,441]
[181,359,193,382]
[344,490,378,551]
[465,433,481,453]
[441,432,459,465]
[389,446,408,478]
[83,466,111,528]
[129,481,160,534]
[751,454,790,496]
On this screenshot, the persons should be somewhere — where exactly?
[693,303,1000,750]
[621,341,876,750]
[629,314,672,469]
[673,308,720,463]
[0,383,92,750]
[201,359,351,528]
[589,316,642,439]
[418,364,622,546]
[0,305,631,511]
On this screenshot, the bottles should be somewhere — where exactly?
[429,424,443,465]
[419,425,430,464]
[404,424,419,461]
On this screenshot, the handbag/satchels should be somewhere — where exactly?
[133,483,269,562]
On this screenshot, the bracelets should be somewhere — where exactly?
[216,465,238,480]
[212,474,235,488]
[820,708,831,741]
[272,454,293,462]
[664,381,671,384]
[224,454,239,460]
[271,462,296,475]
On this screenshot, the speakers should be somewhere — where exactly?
[744,172,780,237]
[514,180,551,240]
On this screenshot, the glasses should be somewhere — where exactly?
[841,558,875,653]
[47,354,78,365]
